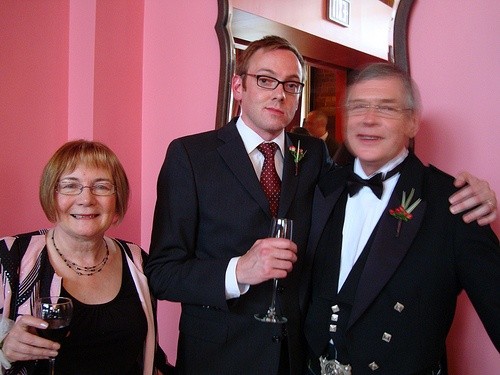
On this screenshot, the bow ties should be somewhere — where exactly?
[348,162,402,200]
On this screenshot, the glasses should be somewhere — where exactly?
[55,181,117,196]
[239,72,304,94]
[345,101,413,117]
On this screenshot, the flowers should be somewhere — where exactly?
[289,139,307,176]
[388,188,421,237]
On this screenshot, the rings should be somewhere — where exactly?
[488,201,495,211]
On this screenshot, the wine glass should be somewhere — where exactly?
[255,217,292,323]
[33,295,76,375]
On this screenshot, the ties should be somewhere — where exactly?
[257,143,282,220]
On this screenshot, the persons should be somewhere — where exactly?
[290,110,339,159]
[301,61,500,375]
[0,139,177,375]
[143,34,500,375]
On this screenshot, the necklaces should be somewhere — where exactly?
[51,227,110,276]
